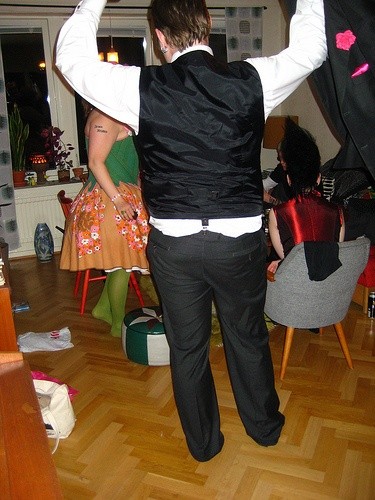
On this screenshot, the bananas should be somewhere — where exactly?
[32,177,35,185]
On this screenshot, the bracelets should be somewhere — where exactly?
[112,194,120,202]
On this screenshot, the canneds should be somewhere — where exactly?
[367,292,375,318]
[15,304,29,312]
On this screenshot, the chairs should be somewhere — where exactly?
[56,189,146,315]
[262,235,371,380]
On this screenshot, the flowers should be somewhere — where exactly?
[41,125,75,170]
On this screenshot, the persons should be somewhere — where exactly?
[60,98,151,337]
[262,118,346,335]
[55,0,327,462]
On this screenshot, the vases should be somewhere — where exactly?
[34,223,54,263]
[57,169,70,183]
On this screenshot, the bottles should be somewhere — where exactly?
[34,223,54,263]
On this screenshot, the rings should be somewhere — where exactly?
[121,211,126,215]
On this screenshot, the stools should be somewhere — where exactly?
[121,305,173,367]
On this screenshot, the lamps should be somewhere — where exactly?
[106,0,120,63]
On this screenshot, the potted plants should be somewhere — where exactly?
[9,104,29,186]
